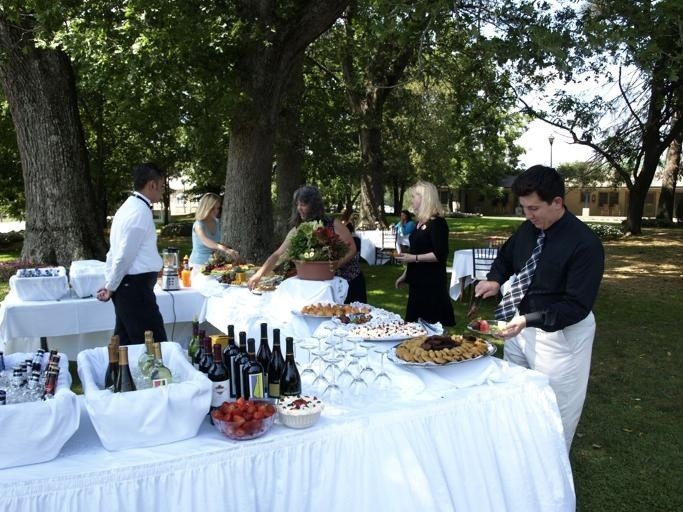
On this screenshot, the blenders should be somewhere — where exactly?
[161,246,182,291]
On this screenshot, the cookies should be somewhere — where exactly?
[396,335,488,364]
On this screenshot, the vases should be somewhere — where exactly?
[291,259,337,281]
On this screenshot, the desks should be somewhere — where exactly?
[449,248,508,302]
[352,229,402,266]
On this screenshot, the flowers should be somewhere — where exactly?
[276,220,351,282]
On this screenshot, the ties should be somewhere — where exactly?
[492,229,546,323]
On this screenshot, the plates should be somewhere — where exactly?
[466,319,510,336]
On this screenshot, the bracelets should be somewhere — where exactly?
[415,255,419,263]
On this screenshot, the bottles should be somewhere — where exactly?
[0,348,62,405]
[182,253,191,287]
[104,331,174,394]
[185,320,302,425]
[17,266,60,277]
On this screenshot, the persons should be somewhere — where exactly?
[187,192,237,264]
[344,212,361,253]
[393,180,456,330]
[391,212,415,262]
[97,166,170,343]
[248,188,367,306]
[474,163,605,458]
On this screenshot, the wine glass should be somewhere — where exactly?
[290,324,397,409]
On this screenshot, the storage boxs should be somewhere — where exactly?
[77,342,212,452]
[1,352,80,471]
[9,265,70,301]
[68,260,107,299]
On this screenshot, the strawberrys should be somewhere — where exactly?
[213,397,275,437]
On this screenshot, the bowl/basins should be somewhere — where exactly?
[276,403,325,429]
[210,406,279,442]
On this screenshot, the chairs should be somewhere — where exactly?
[375,228,398,265]
[465,248,502,318]
[489,236,511,248]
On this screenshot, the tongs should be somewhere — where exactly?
[468,298,483,319]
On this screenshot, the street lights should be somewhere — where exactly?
[546,134,556,168]
